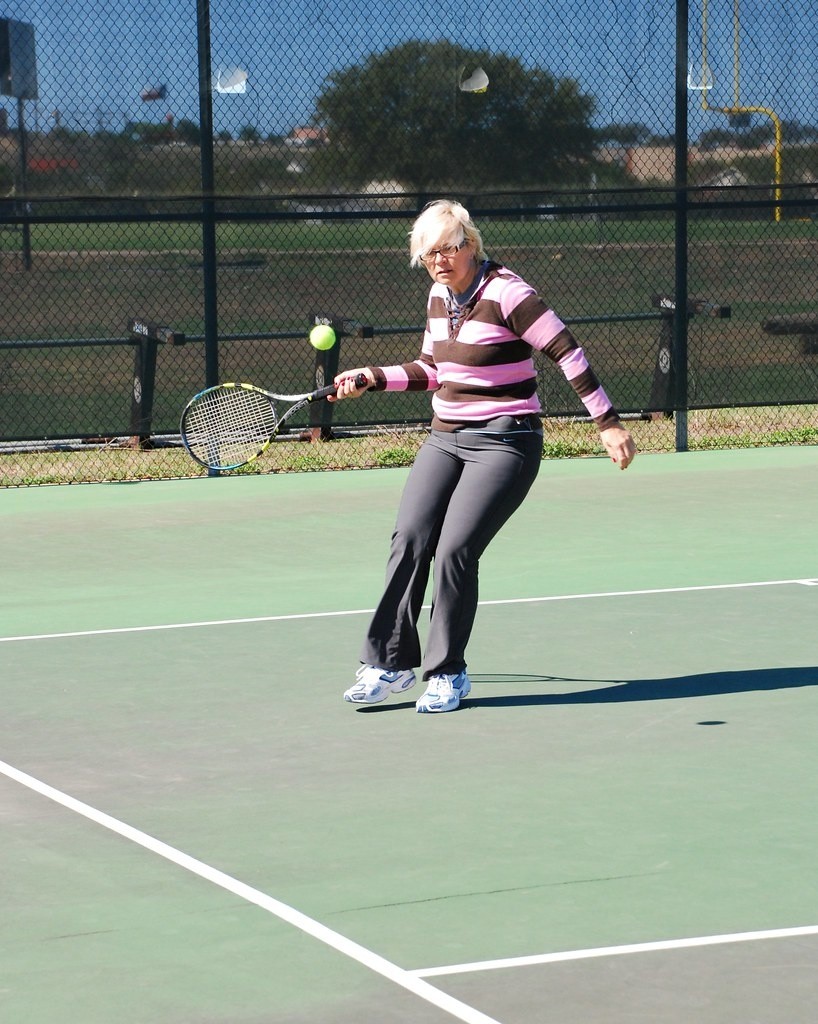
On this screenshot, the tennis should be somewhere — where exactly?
[310,325,336,351]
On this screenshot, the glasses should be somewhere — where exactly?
[418,237,467,261]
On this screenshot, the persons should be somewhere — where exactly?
[325,198,640,714]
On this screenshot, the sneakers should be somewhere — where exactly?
[344,664,417,705]
[416,668,471,712]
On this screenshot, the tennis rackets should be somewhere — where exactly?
[179,373,368,470]
